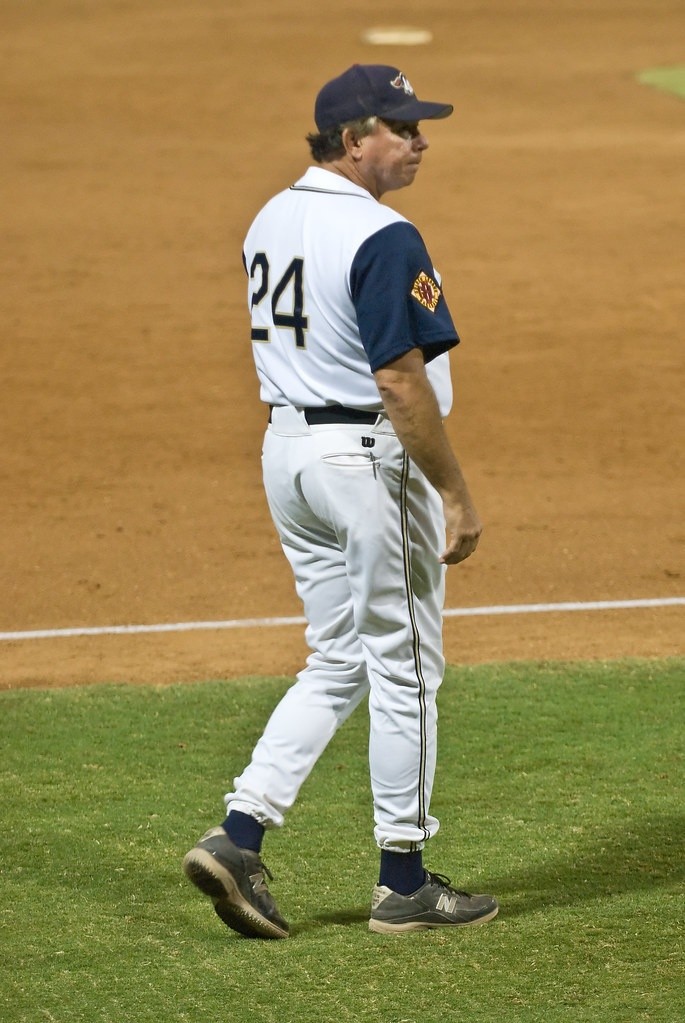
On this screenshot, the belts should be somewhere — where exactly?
[267,404,380,425]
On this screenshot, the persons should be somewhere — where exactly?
[178,63,500,938]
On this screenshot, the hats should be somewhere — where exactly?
[313,63,453,136]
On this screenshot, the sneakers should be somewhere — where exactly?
[181,824,289,939]
[367,867,498,934]
